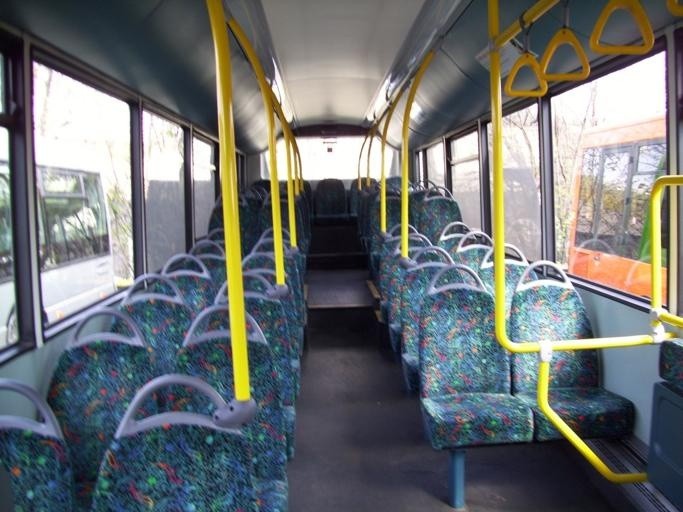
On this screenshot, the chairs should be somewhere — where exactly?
[413,262,536,503]
[177,305,291,510]
[49,305,158,453]
[2,380,73,508]
[512,262,635,444]
[92,374,252,511]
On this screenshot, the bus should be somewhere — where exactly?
[567,111,667,306]
[0,155,118,349]
